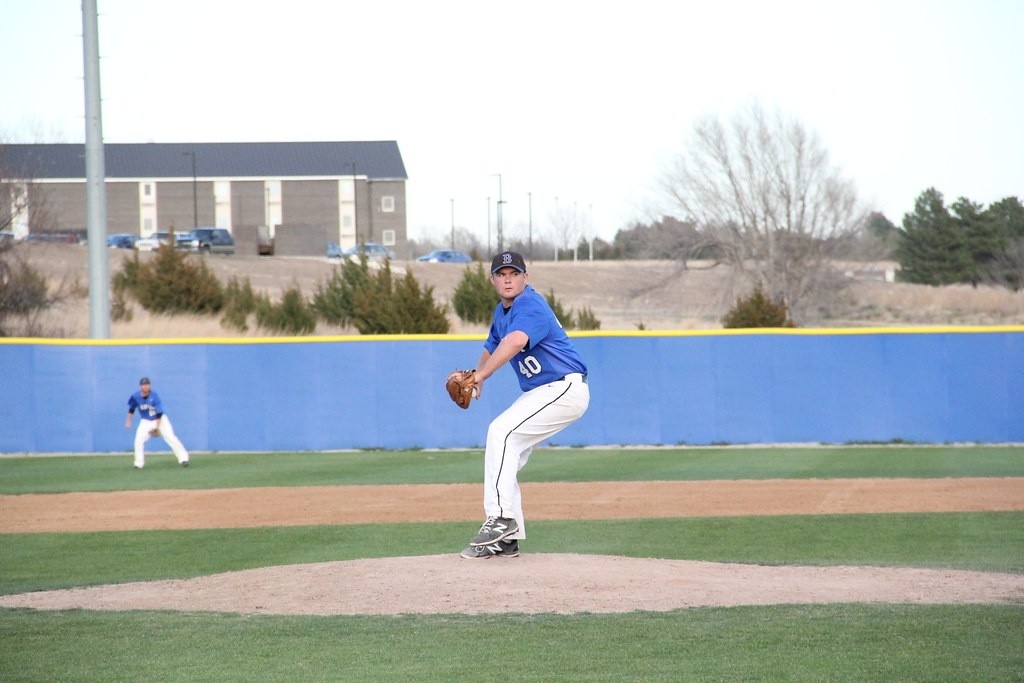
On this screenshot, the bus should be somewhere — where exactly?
[134,231,190,253]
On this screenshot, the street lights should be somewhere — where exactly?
[182,149,198,229]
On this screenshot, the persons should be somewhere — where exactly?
[448,251,589,559]
[126,377,190,469]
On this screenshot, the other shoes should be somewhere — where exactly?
[182,461,189,467]
[134,466,139,469]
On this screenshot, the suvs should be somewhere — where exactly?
[173,227,235,256]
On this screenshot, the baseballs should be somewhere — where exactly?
[471,388,477,398]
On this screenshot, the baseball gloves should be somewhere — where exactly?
[446,369,476,409]
[148,429,161,437]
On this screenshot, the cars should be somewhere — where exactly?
[327,241,344,259]
[342,242,398,262]
[415,249,473,263]
[106,235,140,249]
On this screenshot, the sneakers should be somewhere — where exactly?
[469,516,520,547]
[461,539,520,559]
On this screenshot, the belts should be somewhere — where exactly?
[555,374,589,385]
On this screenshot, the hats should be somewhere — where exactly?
[140,378,151,384]
[491,251,526,275]
